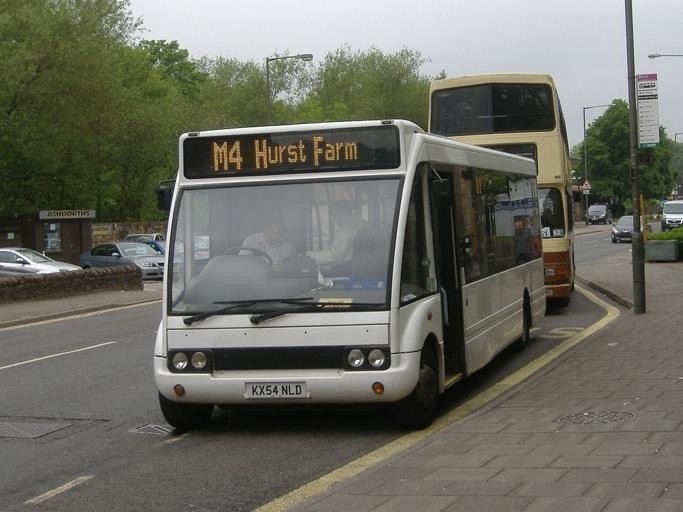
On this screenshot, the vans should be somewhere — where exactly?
[660,200,682,232]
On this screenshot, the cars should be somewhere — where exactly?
[583,203,614,227]
[611,215,652,243]
[80,242,181,281]
[0,245,84,283]
[126,232,212,274]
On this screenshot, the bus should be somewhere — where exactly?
[427,73,576,307]
[150,121,547,431]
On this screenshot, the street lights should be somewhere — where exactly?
[674,133,682,144]
[580,104,617,226]
[647,51,683,64]
[265,53,319,120]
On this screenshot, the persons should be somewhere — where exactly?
[513,215,534,266]
[237,214,300,274]
[306,200,372,269]
